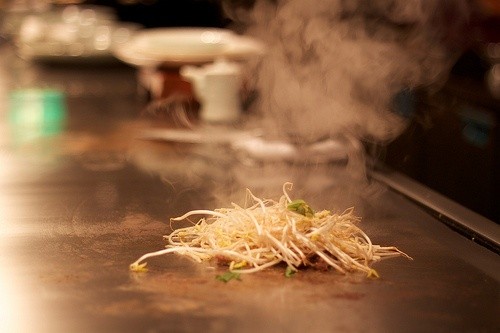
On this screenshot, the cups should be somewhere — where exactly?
[187,66,245,120]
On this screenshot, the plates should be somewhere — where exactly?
[113,28,265,63]
